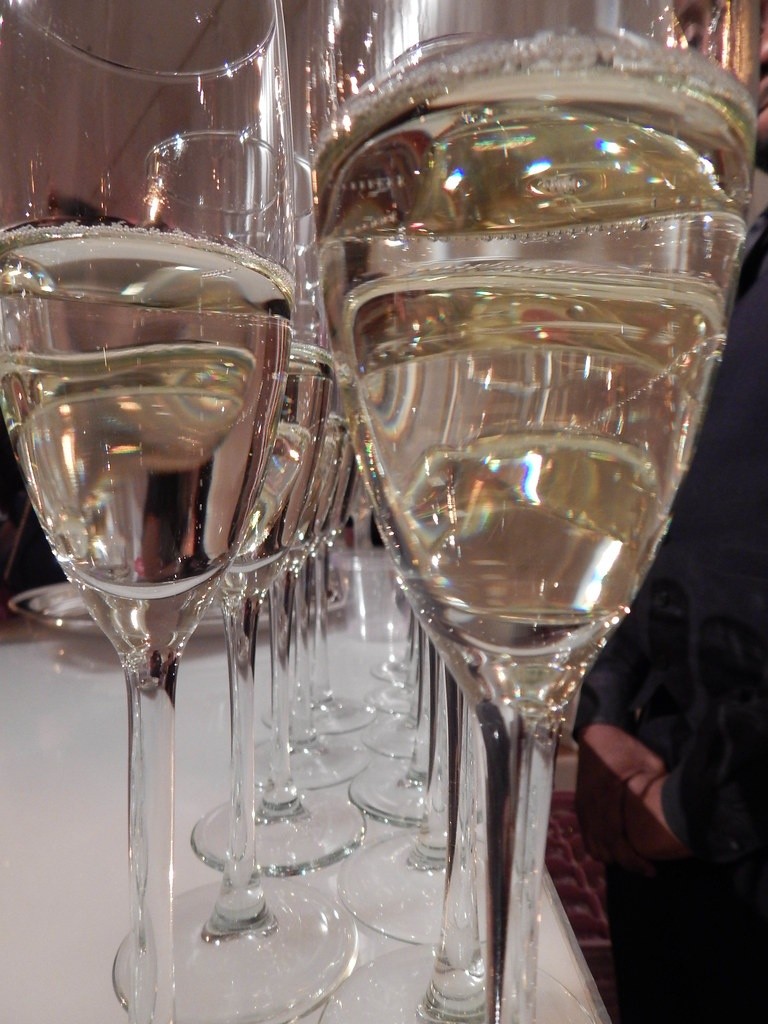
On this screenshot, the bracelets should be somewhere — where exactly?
[639,770,666,800]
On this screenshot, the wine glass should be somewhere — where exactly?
[0,0,757,1024]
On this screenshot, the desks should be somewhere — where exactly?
[0,551,610,1023]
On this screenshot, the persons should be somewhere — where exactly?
[0,191,209,626]
[567,1,768,1024]
